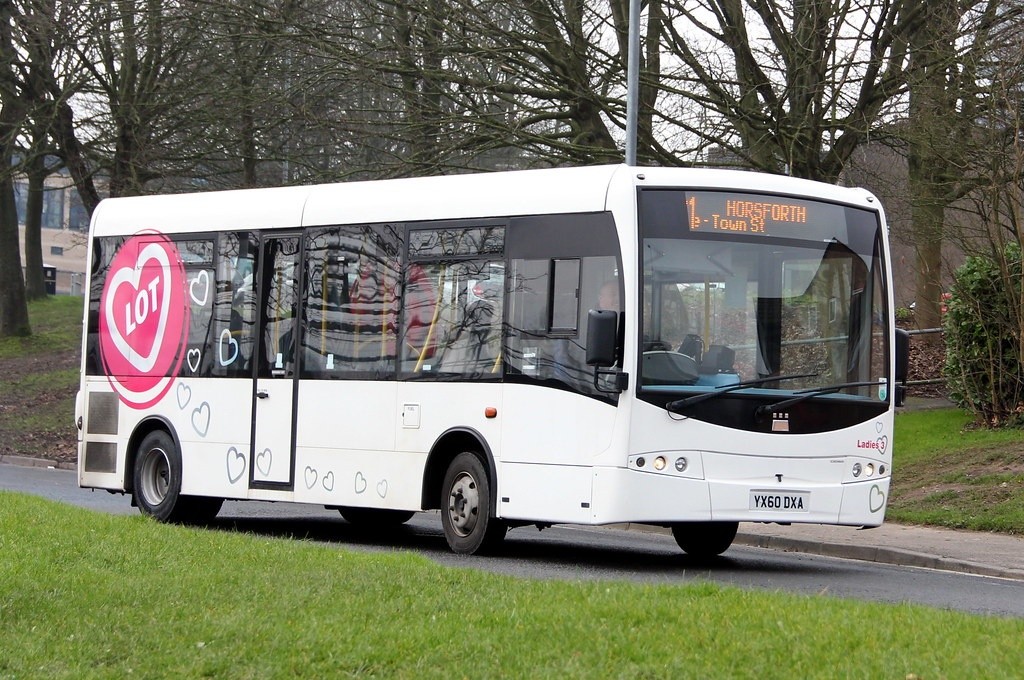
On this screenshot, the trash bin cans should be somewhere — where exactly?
[43,267,56,294]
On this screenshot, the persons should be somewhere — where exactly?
[566,277,622,395]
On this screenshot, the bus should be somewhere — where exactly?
[72,164,911,559]
[11,169,110,298]
